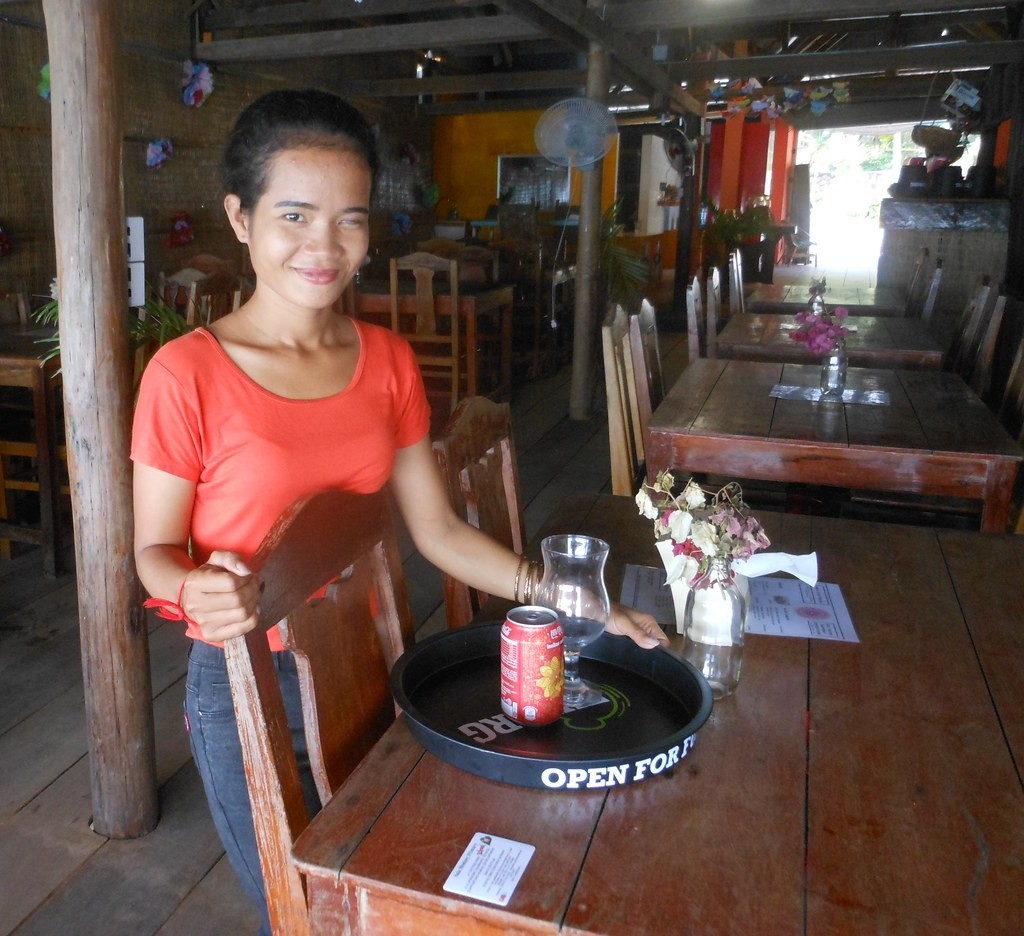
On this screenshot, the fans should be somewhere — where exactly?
[664,128,700,178]
[532,97,619,171]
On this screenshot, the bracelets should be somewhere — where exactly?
[513,554,556,608]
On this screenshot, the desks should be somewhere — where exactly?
[354,280,517,404]
[741,284,908,317]
[0,324,71,492]
[289,491,1023,936]
[717,315,942,370]
[642,358,1024,536]
[722,223,799,283]
[541,259,575,376]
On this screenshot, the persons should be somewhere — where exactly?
[129,87,669,936]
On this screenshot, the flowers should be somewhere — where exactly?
[789,300,851,356]
[635,471,771,585]
[808,281,826,294]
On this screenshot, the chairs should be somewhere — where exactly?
[683,244,746,361]
[0,251,253,582]
[598,298,782,506]
[432,396,530,629]
[334,232,546,413]
[909,248,1024,444]
[224,485,416,936]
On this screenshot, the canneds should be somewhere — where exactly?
[500,605,564,726]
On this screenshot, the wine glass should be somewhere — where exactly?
[534,534,610,708]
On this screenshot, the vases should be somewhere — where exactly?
[809,293,828,315]
[683,558,748,700]
[819,345,849,399]
